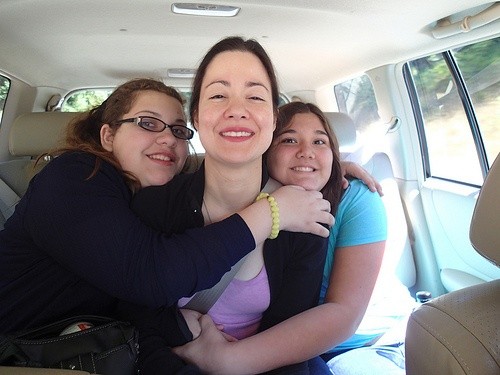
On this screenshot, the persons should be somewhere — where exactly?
[156,35,331,375]
[0,77,385,375]
[168,102,422,375]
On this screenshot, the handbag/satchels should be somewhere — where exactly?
[10,315,142,375]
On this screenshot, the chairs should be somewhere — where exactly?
[7,113,500,375]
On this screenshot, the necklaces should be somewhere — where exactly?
[203,197,213,224]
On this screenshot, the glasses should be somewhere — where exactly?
[112,116,194,140]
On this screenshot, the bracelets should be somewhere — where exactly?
[254,191,280,240]
